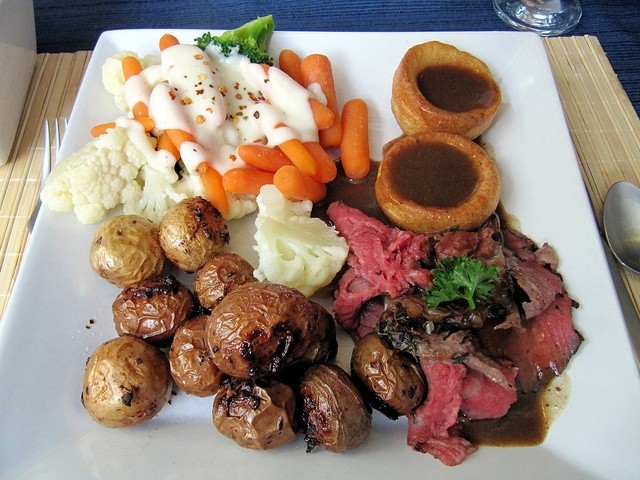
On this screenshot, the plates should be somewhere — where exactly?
[0,27,640,480]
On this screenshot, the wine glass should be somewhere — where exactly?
[490,0,583,35]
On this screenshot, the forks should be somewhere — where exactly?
[27,116,67,232]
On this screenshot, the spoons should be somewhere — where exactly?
[601,179,640,279]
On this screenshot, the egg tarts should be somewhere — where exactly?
[375,132,501,237]
[390,42,501,141]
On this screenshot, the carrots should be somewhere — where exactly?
[300,53,343,147]
[272,165,327,203]
[303,142,336,184]
[165,126,208,177]
[278,138,321,176]
[341,99,370,181]
[270,67,335,131]
[90,118,156,136]
[279,48,301,87]
[160,33,179,53]
[196,161,227,216]
[236,145,295,175]
[221,170,275,197]
[122,56,154,121]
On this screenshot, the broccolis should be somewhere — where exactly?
[193,13,274,73]
[254,183,315,224]
[252,217,349,299]
[37,128,188,225]
[100,49,165,119]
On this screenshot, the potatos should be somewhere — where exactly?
[158,132,181,162]
